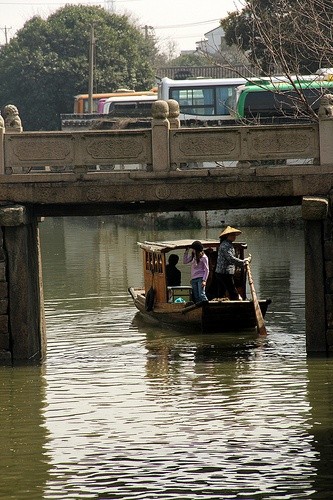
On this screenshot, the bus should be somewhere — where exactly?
[75,68,333,168]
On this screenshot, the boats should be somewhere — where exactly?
[127,239,270,332]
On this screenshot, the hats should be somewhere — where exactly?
[219,226,242,238]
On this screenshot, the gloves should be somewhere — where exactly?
[243,258,250,262]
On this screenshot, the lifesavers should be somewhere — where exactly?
[144,287,155,313]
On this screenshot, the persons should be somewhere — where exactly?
[215,225,250,301]
[183,241,209,301]
[166,253,181,286]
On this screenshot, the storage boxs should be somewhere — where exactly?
[167,286,193,304]
